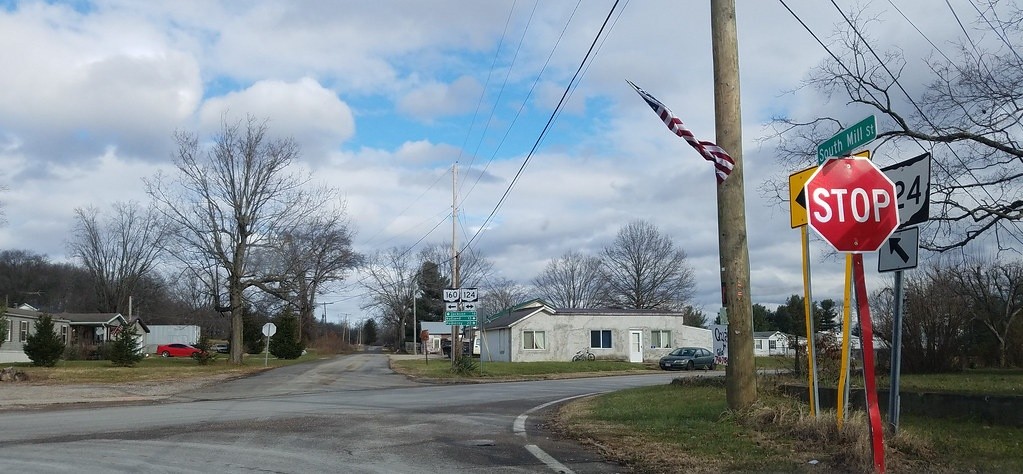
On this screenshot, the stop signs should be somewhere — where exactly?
[805,156,901,254]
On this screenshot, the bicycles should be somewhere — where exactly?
[572,347,595,361]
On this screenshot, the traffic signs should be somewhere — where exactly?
[443,289,478,326]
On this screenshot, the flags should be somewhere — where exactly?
[625,78,735,185]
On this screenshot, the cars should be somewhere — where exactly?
[659,347,716,371]
[157,343,203,358]
[211,344,228,353]
